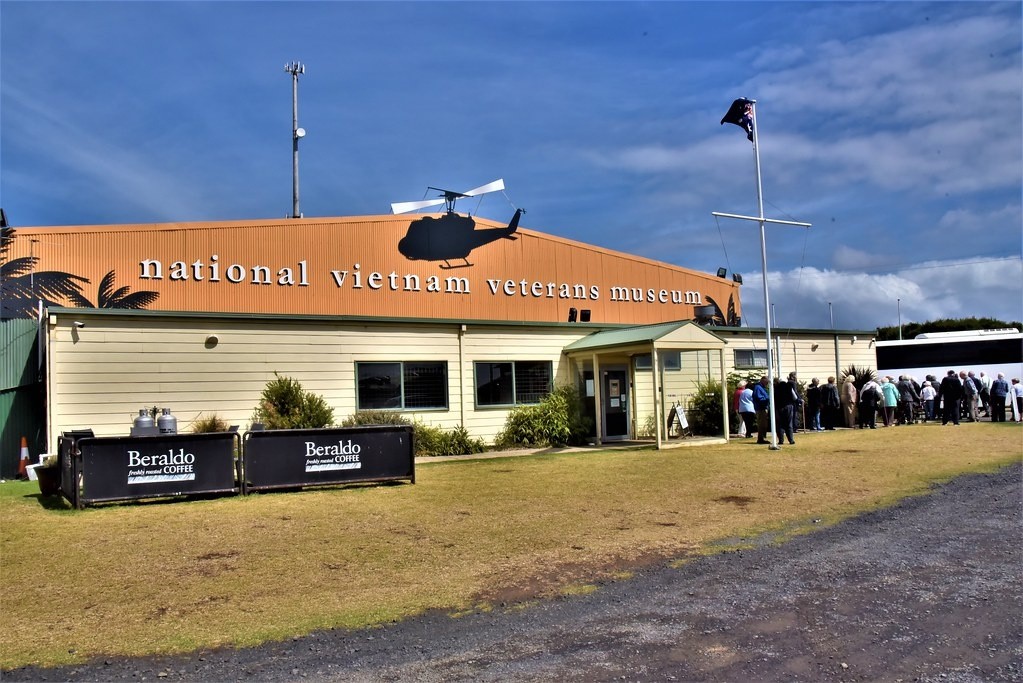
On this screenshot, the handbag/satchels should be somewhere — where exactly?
[1005,392,1011,406]
[875,404,880,411]
[940,400,944,408]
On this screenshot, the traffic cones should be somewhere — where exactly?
[18,437,32,479]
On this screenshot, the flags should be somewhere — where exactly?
[721,97,754,142]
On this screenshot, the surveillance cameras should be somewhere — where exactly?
[872,337,876,342]
[73,321,85,328]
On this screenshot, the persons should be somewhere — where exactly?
[1010,378,1023,421]
[859,370,1008,429]
[774,372,804,444]
[734,377,770,443]
[841,375,858,427]
[806,377,840,430]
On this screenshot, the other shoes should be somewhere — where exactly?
[810,428,817,431]
[942,423,947,425]
[790,441,794,444]
[757,440,770,444]
[954,423,960,426]
[778,442,783,444]
[746,435,753,438]
[737,434,746,437]
[793,428,798,432]
[982,414,989,417]
[817,429,826,432]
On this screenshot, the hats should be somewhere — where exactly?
[736,380,748,387]
[924,381,931,385]
[879,378,889,382]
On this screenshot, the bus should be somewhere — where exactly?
[876,327,1023,413]
